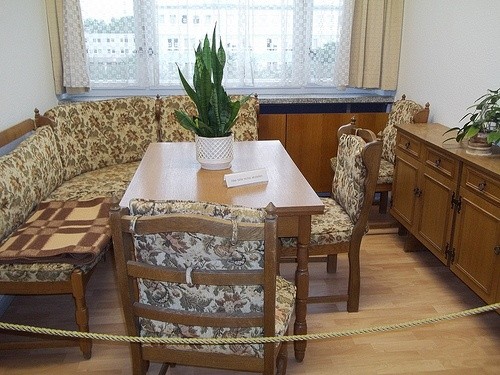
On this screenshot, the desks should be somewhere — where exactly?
[119,140,324,362]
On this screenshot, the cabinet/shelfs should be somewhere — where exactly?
[390,123,500,315]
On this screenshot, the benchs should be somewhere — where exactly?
[0,92,261,360]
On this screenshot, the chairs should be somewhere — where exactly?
[330,95,430,214]
[109,198,297,375]
[278,115,384,312]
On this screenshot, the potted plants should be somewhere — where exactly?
[442,88,500,148]
[174,21,254,170]
